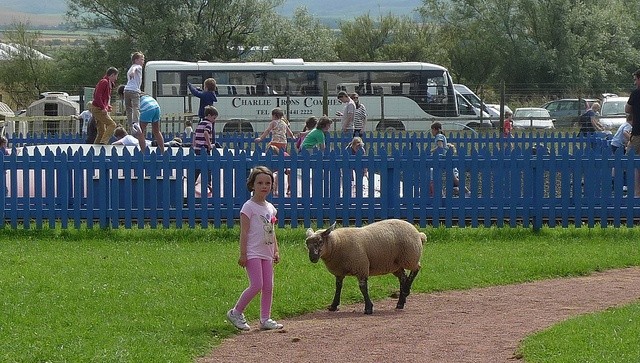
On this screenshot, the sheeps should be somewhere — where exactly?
[304,218,428,316]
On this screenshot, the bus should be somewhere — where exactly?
[143,57,461,133]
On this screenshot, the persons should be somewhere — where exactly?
[335,91,356,181]
[610,115,632,199]
[429,121,447,197]
[296,115,318,150]
[504,111,515,146]
[87,115,100,143]
[0,136,17,196]
[349,93,368,152]
[111,126,152,146]
[185,120,193,138]
[181,77,218,123]
[344,137,368,182]
[123,50,145,134]
[118,84,164,154]
[227,165,284,332]
[91,65,119,144]
[254,109,296,156]
[298,115,331,152]
[194,104,219,194]
[447,143,471,197]
[585,102,603,132]
[624,69,640,198]
[71,101,95,137]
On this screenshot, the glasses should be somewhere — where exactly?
[353,96,359,98]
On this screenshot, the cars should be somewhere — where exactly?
[512,107,557,130]
[598,95,631,133]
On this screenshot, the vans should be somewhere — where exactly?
[540,98,603,129]
[453,88,492,130]
[339,83,505,126]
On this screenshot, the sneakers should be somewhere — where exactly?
[227,308,251,331]
[258,318,283,329]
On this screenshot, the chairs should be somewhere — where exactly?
[373,85,377,93]
[215,87,218,96]
[232,86,237,94]
[391,85,401,94]
[172,86,177,95]
[355,86,359,93]
[342,86,346,91]
[251,85,255,95]
[337,86,340,92]
[269,86,274,96]
[246,86,250,94]
[376,86,383,94]
[227,86,232,95]
